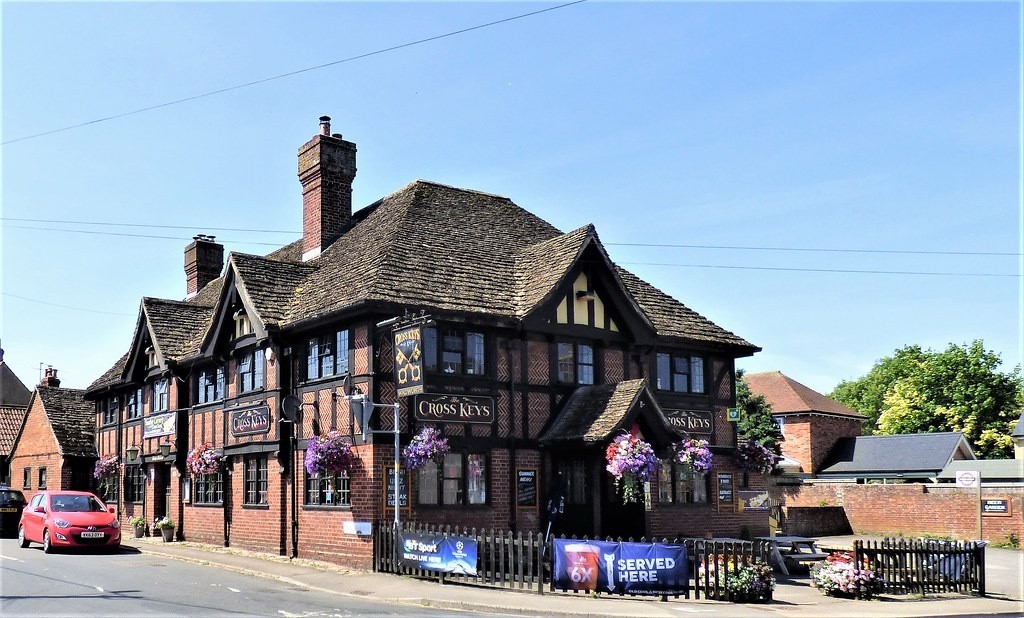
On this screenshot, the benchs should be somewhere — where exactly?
[785,553,830,575]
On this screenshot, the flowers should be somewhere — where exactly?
[187,444,222,477]
[737,440,775,475]
[303,431,356,478]
[699,553,776,595]
[93,453,120,480]
[604,432,661,505]
[402,427,451,470]
[672,437,714,477]
[809,550,886,596]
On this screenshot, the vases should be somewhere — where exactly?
[703,586,773,603]
[831,589,856,599]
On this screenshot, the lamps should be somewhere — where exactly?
[159,436,177,458]
[125,442,143,462]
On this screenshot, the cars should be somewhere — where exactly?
[0,485,27,536]
[17,491,121,554]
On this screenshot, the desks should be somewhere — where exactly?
[680,537,754,546]
[752,536,820,577]
